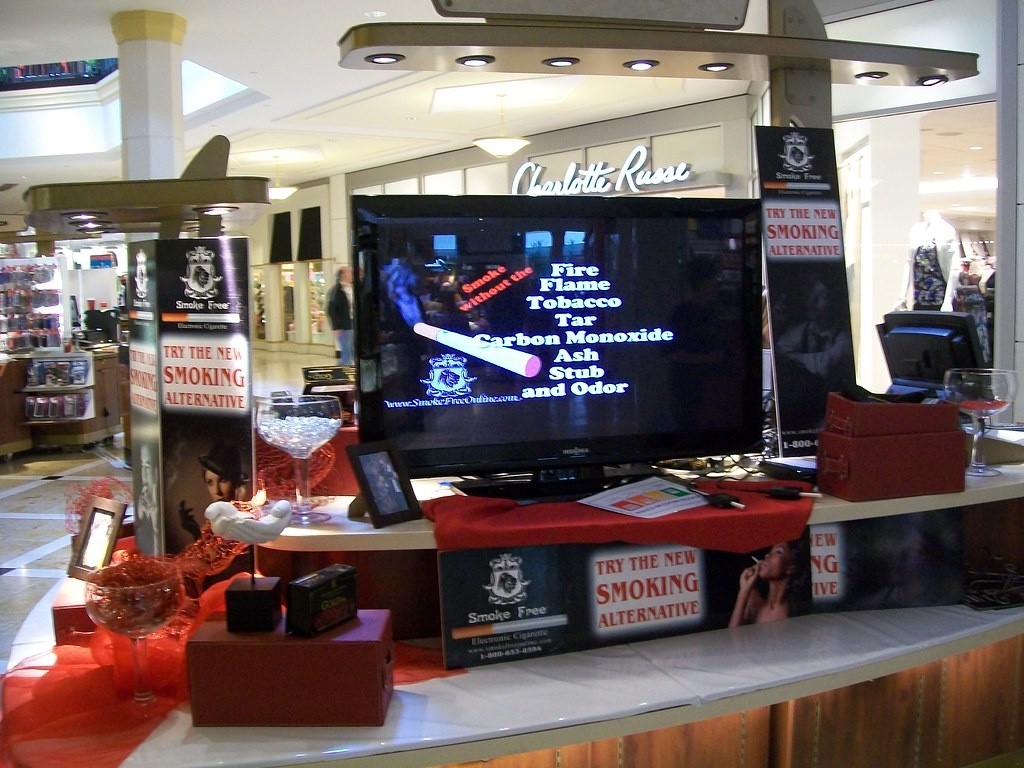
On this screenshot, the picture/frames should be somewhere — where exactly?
[345,439,423,529]
[66,494,128,583]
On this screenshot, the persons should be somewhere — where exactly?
[204,499,292,544]
[671,255,745,430]
[366,455,408,514]
[178,438,250,542]
[328,267,355,367]
[911,209,961,312]
[728,540,800,629]
[775,269,850,431]
[978,266,995,294]
[884,517,949,610]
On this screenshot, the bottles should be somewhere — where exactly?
[0,289,60,308]
[0,332,61,351]
[0,309,60,333]
[70,296,81,327]
[25,394,90,417]
[28,363,87,386]
[63,338,72,352]
[0,265,56,284]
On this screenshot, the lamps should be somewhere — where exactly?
[471,89,532,159]
[268,155,299,201]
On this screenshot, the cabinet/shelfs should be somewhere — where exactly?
[31,353,122,452]
[11,351,96,427]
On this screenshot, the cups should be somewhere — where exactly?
[122,331,130,343]
[101,303,107,307]
[87,300,95,310]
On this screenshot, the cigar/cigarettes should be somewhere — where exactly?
[763,181,833,192]
[752,556,760,564]
[129,311,153,320]
[452,614,567,639]
[162,313,241,324]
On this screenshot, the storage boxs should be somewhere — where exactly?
[818,429,965,503]
[823,391,960,438]
[284,563,358,639]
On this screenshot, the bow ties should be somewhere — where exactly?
[810,329,829,346]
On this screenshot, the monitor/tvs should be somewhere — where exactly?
[351,196,766,501]
[875,310,993,403]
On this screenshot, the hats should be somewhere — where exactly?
[960,258,973,266]
[198,442,251,484]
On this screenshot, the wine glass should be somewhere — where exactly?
[257,395,342,526]
[944,369,1016,478]
[85,562,185,719]
